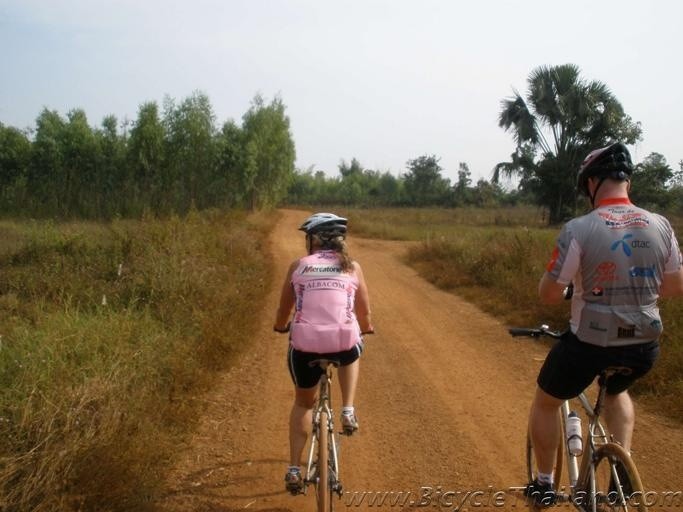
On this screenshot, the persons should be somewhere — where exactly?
[274,211,375,490]
[524,141,683,509]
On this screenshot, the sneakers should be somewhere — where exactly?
[286,469,302,488]
[339,412,360,430]
[608,460,633,504]
[523,478,554,502]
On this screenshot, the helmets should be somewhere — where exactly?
[575,141,632,198]
[299,212,348,237]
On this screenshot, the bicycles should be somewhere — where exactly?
[274,322,374,510]
[507,325,649,512]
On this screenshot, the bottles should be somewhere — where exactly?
[566,410,583,457]
